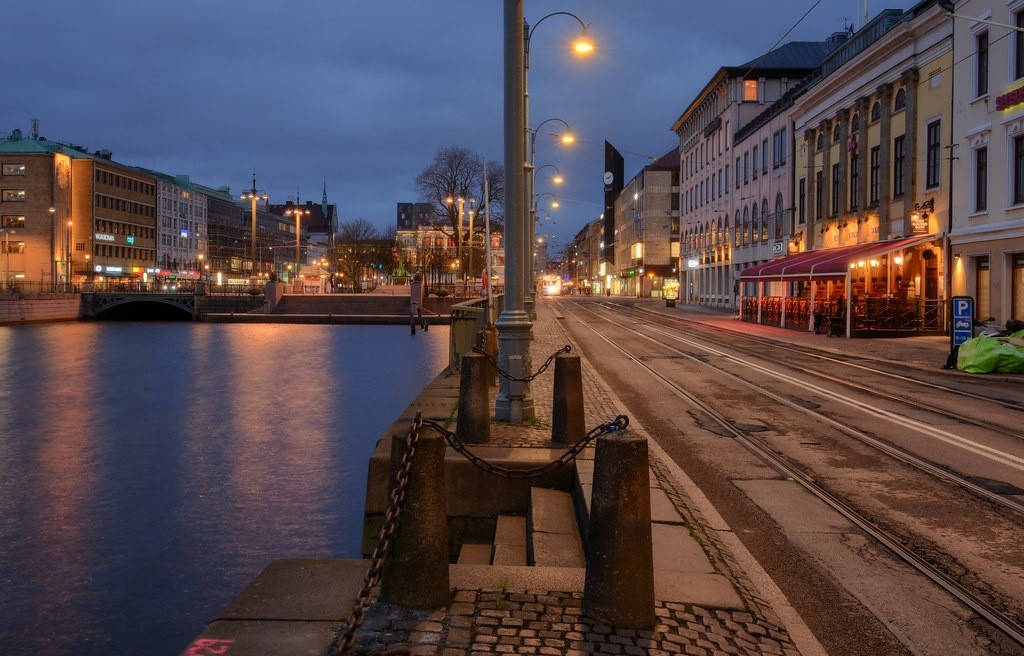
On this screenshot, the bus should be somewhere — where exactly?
[541,276,562,296]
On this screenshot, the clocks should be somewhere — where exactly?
[603,171,614,185]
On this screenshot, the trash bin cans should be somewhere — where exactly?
[827,316,845,337]
[607,288,611,296]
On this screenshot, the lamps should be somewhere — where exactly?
[837,218,850,229]
[881,255,888,269]
[955,251,962,259]
[858,213,870,224]
[788,231,803,247]
[907,197,934,221]
[821,223,832,233]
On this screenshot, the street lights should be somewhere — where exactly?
[241,193,268,272]
[198,255,203,279]
[468,197,476,279]
[285,206,310,277]
[204,264,209,281]
[85,255,90,281]
[447,196,465,280]
[523,118,576,320]
[5,217,25,283]
[494,11,595,426]
[49,207,55,291]
